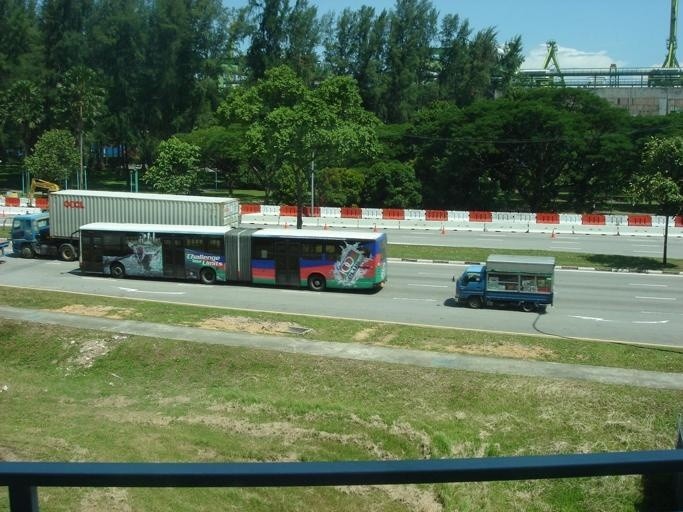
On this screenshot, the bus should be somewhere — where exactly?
[69,221,388,293]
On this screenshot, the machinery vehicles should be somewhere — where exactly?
[27,177,60,208]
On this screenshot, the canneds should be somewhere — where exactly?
[338,249,365,281]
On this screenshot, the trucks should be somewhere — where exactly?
[2,187,242,260]
[451,255,559,313]
